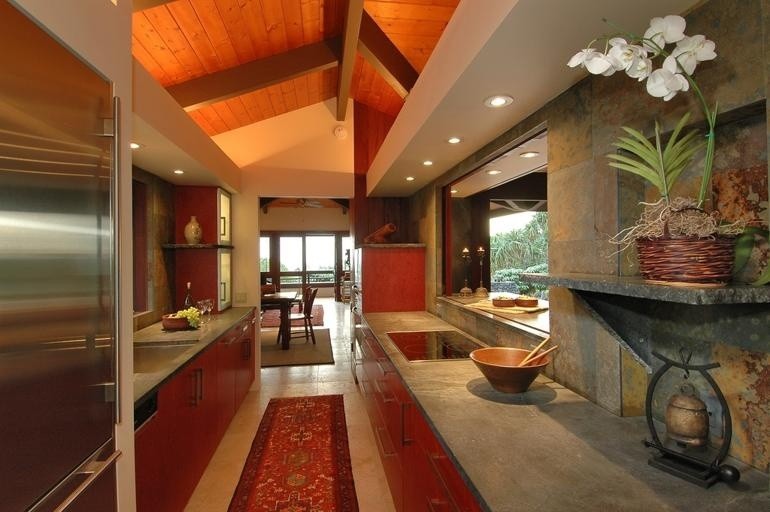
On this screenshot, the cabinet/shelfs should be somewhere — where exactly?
[356,318,485,512]
[176,185,232,312]
[134,313,255,512]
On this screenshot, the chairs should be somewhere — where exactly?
[276,284,317,345]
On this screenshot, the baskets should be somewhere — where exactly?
[635,207,736,288]
[493,300,538,307]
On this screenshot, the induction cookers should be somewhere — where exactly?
[386,330,487,367]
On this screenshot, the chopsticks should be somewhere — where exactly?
[518,336,557,366]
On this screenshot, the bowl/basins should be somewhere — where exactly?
[493,299,516,307]
[162,314,189,329]
[469,345,549,394]
[515,298,538,307]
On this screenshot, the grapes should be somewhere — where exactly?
[176,306,201,329]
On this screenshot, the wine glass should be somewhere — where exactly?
[205,299,215,321]
[196,300,208,324]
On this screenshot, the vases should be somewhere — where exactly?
[184,215,203,245]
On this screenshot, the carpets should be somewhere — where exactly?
[261,328,334,366]
[261,304,323,326]
[228,394,359,512]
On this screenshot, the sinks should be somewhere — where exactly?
[133,340,199,374]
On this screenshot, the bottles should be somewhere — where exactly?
[185,282,194,308]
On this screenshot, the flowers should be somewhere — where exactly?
[566,14,723,208]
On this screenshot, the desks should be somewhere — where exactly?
[261,292,297,350]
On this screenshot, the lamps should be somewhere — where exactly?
[476,247,489,297]
[460,248,473,296]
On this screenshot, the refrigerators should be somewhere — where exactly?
[0,4,124,510]
